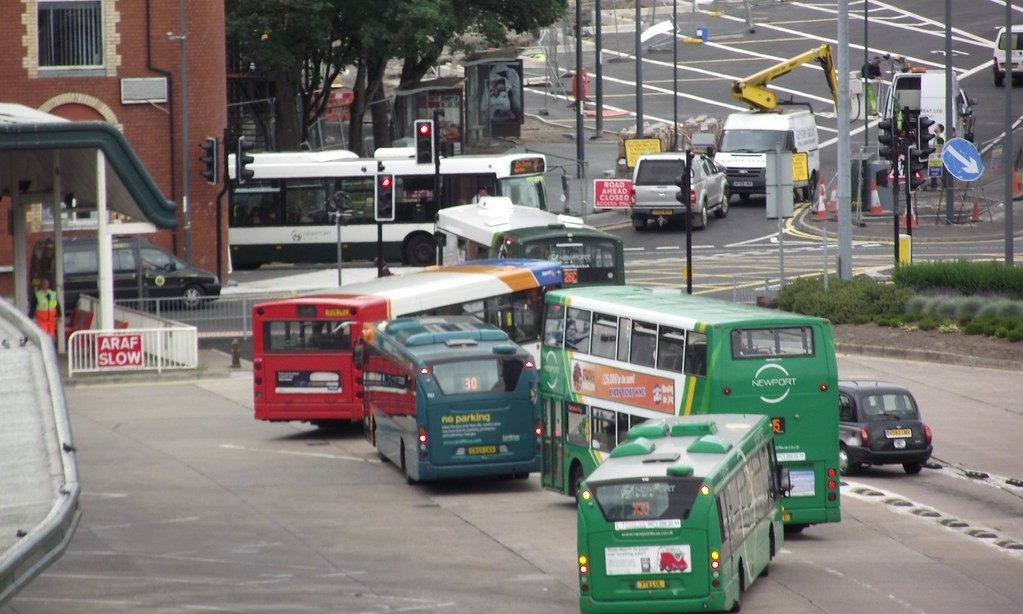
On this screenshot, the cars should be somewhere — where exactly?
[837,380,933,477]
[960,87,978,142]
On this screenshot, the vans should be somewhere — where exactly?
[881,68,971,167]
[709,105,818,201]
[994,24,1023,87]
[29,237,221,310]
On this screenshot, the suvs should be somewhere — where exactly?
[630,152,730,233]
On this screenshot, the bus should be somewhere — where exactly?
[578,414,791,614]
[352,315,539,486]
[253,257,563,434]
[434,196,625,335]
[228,148,568,268]
[535,285,842,538]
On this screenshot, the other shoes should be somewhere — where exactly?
[923,184,937,192]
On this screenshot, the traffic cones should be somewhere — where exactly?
[867,180,885,216]
[900,195,918,229]
[813,172,837,221]
[1014,168,1021,201]
[970,201,983,222]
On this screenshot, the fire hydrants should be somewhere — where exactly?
[228,339,241,368]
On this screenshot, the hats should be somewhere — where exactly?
[478,184,488,190]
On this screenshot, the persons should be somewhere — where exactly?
[926,123,946,187]
[861,57,884,116]
[32,275,57,343]
[471,186,491,205]
[247,198,330,225]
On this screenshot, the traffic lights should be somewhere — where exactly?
[235,136,254,186]
[415,120,434,164]
[917,116,935,161]
[907,144,925,194]
[200,136,219,185]
[375,174,394,222]
[877,117,893,161]
